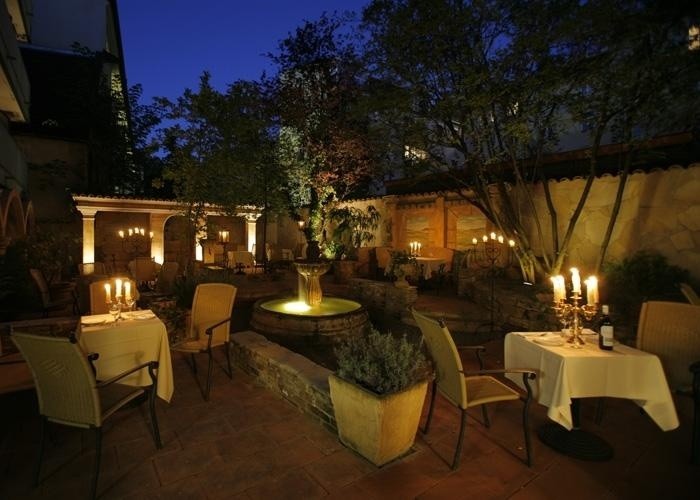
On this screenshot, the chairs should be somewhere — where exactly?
[409,304,540,474]
[633,297,700,473]
[26,254,184,316]
[167,281,239,402]
[7,329,164,499]
[433,247,467,298]
[673,278,699,308]
[372,245,397,282]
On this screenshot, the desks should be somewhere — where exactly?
[382,252,448,297]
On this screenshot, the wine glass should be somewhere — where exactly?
[560,319,569,334]
[109,298,121,327]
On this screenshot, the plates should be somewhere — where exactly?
[120,311,155,320]
[534,337,564,346]
[81,315,106,324]
[586,336,619,347]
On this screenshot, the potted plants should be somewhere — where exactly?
[327,201,384,285]
[324,324,447,470]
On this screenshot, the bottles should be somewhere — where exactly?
[599,305,614,351]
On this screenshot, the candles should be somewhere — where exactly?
[551,270,600,306]
[409,241,422,254]
[117,225,154,239]
[471,232,516,247]
[218,230,230,243]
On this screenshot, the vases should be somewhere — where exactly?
[305,239,321,260]
